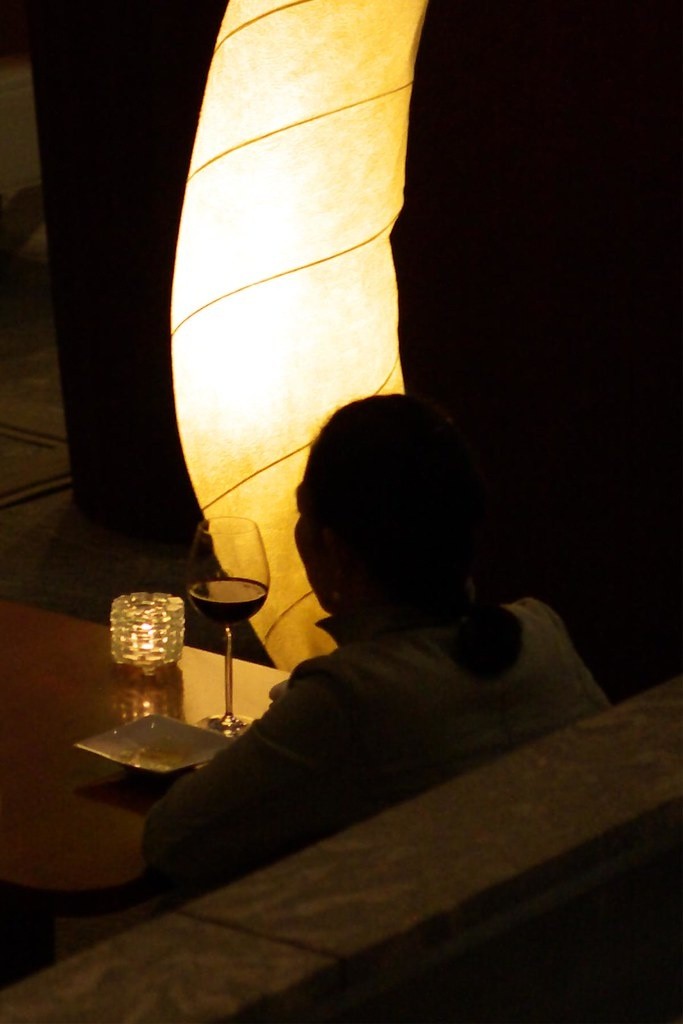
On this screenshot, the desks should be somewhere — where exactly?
[1,602,292,987]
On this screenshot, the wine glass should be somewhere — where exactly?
[185,517,271,737]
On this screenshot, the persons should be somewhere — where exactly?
[138,392,616,896]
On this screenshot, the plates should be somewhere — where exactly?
[72,713,236,774]
